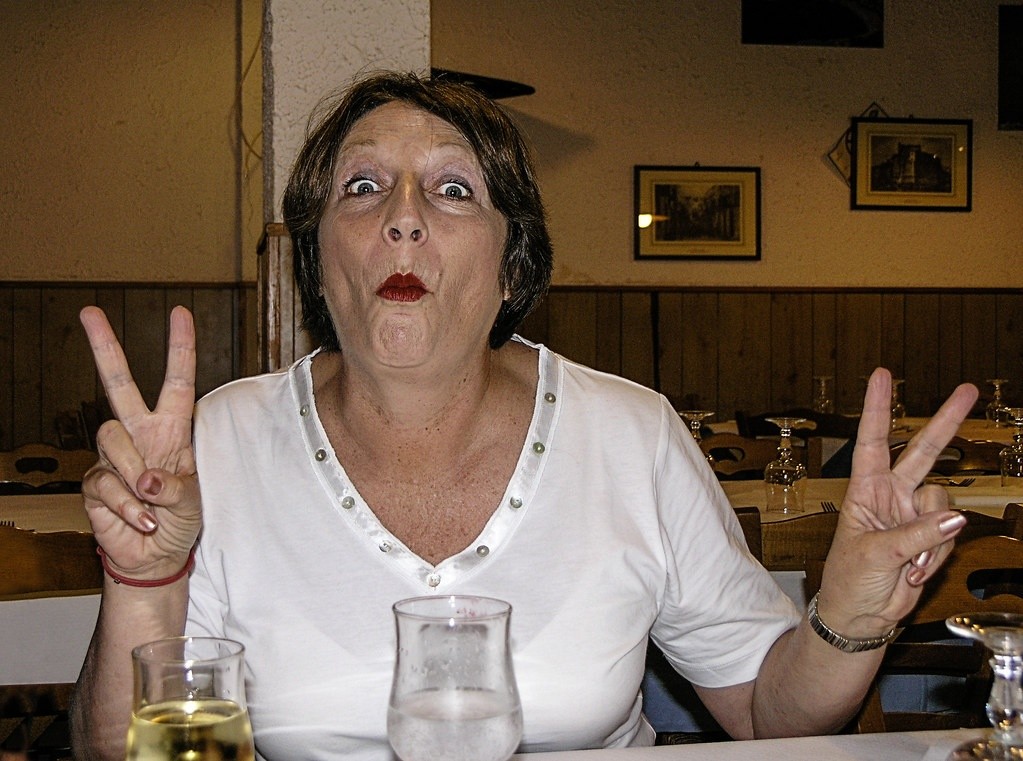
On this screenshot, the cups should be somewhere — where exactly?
[388,595,524,761]
[126,636,256,761]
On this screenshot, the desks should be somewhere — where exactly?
[687,414,1023,525]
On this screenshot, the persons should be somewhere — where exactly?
[70,70,980,761]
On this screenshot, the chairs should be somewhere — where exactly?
[733,498,1023,734]
[0,444,102,495]
[699,406,866,482]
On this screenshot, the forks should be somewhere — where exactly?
[821,501,837,513]
[933,477,975,487]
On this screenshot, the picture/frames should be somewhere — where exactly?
[634,164,762,263]
[849,114,973,214]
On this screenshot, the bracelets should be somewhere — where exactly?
[96,546,194,587]
[808,588,905,653]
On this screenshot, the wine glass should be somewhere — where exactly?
[996,407,1023,488]
[763,417,807,514]
[944,609,1023,761]
[813,375,836,413]
[889,379,906,434]
[679,409,716,467]
[985,378,1009,428]
